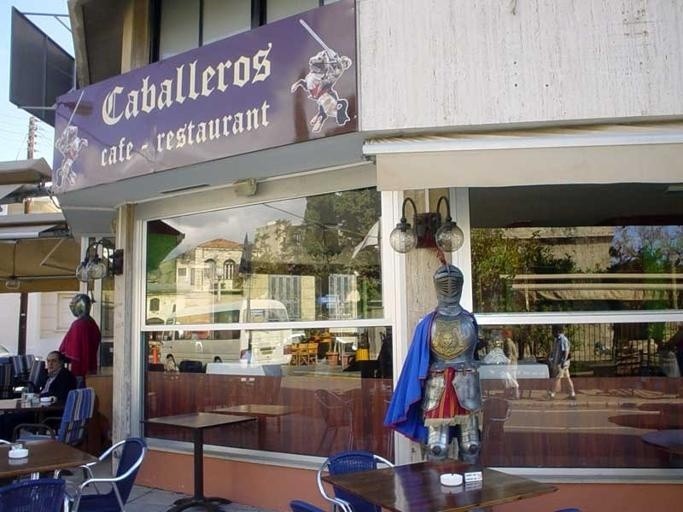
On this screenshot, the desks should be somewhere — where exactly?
[642,429,682,458]
[321,456,559,512]
[139,412,258,512]
[214,402,301,433]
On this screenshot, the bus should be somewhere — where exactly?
[160,297,294,375]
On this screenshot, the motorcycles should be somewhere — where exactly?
[590,339,614,359]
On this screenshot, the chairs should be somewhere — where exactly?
[1,354,147,512]
[314,385,512,465]
[290,449,395,512]
[290,343,319,369]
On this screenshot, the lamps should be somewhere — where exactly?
[390,195,464,254]
[76,238,123,283]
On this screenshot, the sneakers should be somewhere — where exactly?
[541,390,557,400]
[562,394,576,400]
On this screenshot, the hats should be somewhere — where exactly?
[502,328,512,338]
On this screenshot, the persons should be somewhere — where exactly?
[497,325,580,401]
[0,350,77,441]
